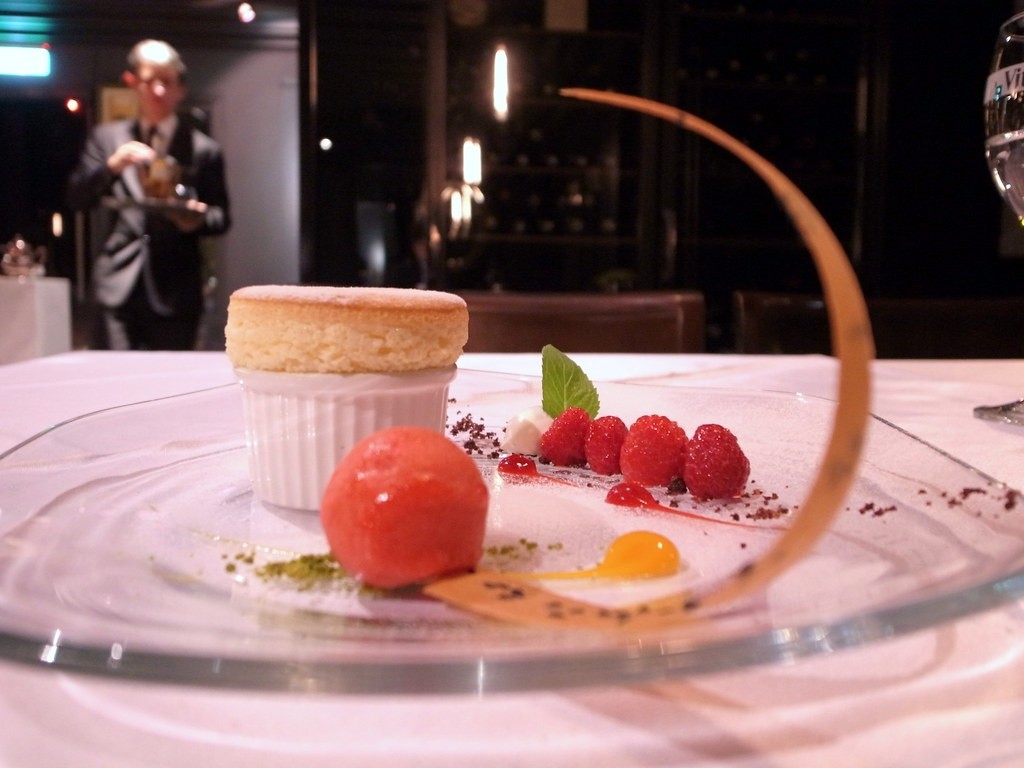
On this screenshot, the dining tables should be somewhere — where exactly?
[0,349,1024,768]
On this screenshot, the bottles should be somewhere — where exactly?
[441,80,620,293]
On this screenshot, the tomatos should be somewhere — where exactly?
[320,425,489,588]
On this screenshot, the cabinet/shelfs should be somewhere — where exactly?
[421,0,894,292]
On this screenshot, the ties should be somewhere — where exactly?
[145,124,157,147]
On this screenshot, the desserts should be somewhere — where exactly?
[225,285,469,511]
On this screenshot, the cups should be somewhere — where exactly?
[232,364,459,511]
[138,156,178,197]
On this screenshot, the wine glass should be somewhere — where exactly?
[970,12,1023,426]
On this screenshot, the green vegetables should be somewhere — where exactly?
[542,343,600,421]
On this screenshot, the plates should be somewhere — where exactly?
[0,376,1023,700]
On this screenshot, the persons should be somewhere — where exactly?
[70,40,231,350]
